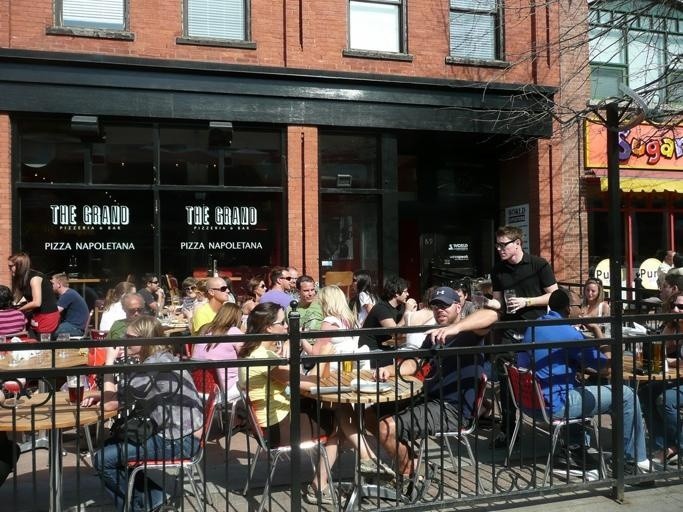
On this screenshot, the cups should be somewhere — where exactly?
[504,289,518,314]
[1,387,17,408]
[470,286,483,308]
[339,350,354,373]
[66,376,84,405]
[0,333,70,366]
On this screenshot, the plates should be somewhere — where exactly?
[352,381,391,394]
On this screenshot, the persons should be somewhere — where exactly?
[0,225,683,512]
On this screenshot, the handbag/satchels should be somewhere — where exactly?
[110,413,158,446]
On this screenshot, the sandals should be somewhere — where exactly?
[650,453,679,465]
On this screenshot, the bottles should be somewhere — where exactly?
[67,255,80,279]
[636,334,668,374]
[206,252,218,278]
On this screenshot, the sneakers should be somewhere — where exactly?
[605,457,656,489]
[306,483,345,505]
[403,460,438,504]
[356,457,398,481]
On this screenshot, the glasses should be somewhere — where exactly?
[570,304,583,310]
[496,239,515,248]
[670,302,683,311]
[272,319,286,326]
[280,276,292,281]
[214,285,230,292]
[8,264,15,269]
[149,280,159,285]
[184,287,197,292]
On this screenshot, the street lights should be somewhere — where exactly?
[583,79,656,503]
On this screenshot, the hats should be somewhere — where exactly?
[429,286,461,306]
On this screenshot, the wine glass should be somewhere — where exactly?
[161,295,187,329]
